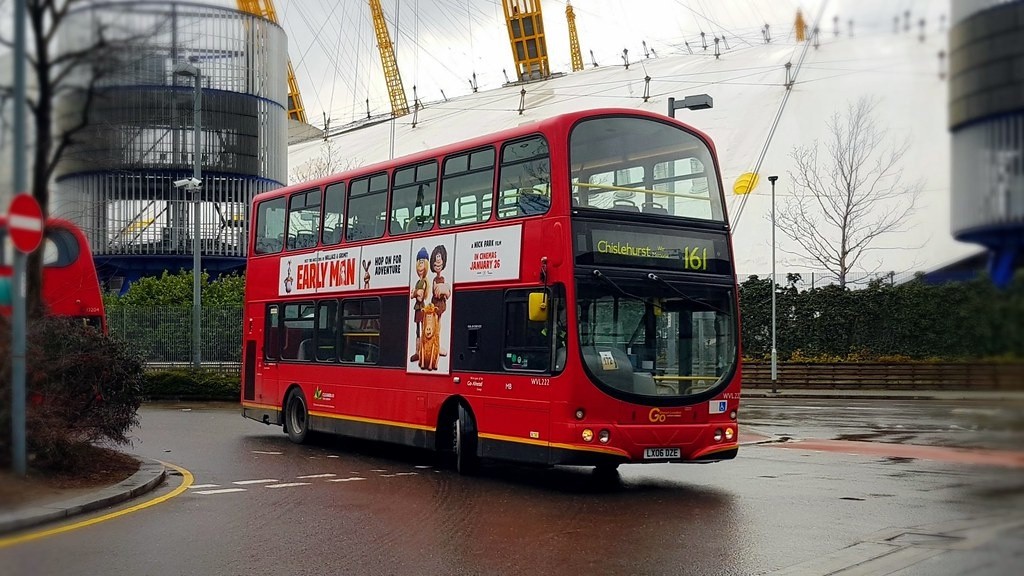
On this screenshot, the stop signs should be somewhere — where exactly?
[6,194,44,255]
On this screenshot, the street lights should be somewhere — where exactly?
[168,61,204,370]
[667,92,714,217]
[768,175,779,394]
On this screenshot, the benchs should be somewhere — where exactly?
[269,326,338,362]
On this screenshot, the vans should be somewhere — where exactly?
[0,212,109,336]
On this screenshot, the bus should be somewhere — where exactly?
[236,106,744,477]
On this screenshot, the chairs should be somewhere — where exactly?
[516,188,667,219]
[259,210,456,254]
